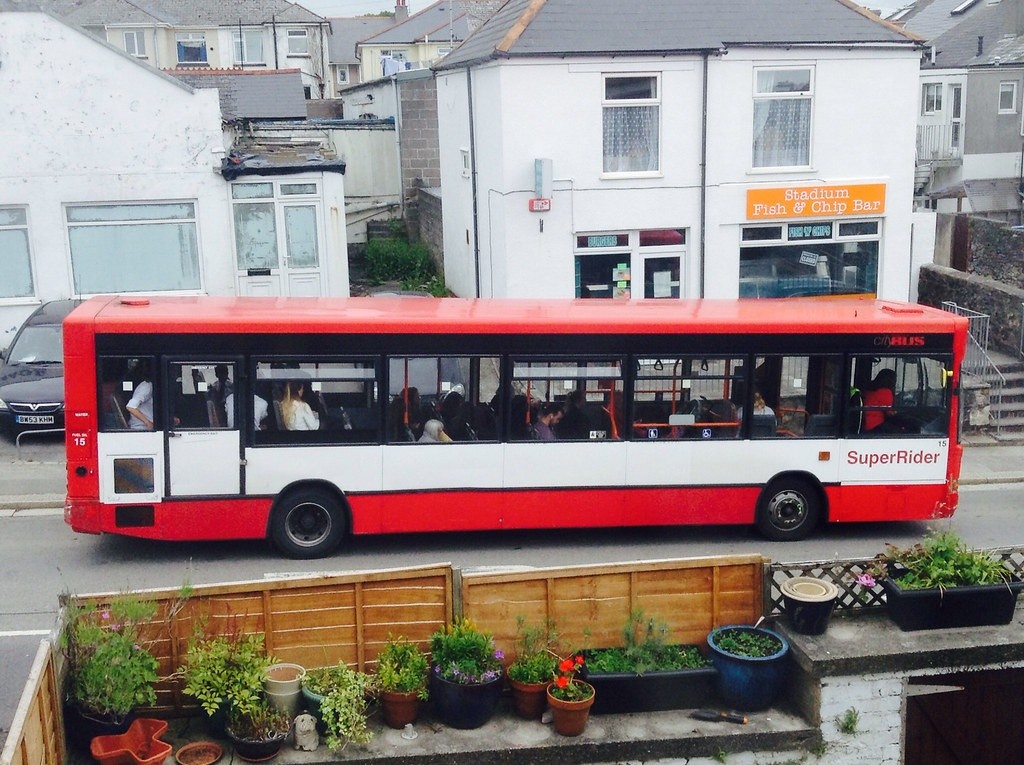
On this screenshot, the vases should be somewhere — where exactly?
[780,577,838,635]
[89,718,171,765]
[881,564,1023,633]
[262,663,305,720]
[548,680,595,737]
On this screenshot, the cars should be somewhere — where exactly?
[0,299,88,439]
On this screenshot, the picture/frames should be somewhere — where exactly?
[74,705,135,759]
[430,661,502,730]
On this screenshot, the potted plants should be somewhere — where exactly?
[706,625,789,712]
[573,610,718,714]
[181,617,277,737]
[301,671,375,755]
[222,699,289,761]
[507,615,559,721]
[377,634,429,729]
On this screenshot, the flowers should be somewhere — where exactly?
[855,532,1011,590]
[56,582,193,720]
[430,617,505,684]
[553,656,588,700]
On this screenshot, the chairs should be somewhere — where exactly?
[110,372,864,441]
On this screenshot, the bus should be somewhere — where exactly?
[63,296,970,560]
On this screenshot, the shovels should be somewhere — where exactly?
[690,709,748,724]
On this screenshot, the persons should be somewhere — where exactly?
[192,368,205,394]
[224,391,269,430]
[418,419,453,442]
[861,368,897,435]
[125,368,180,430]
[398,386,429,442]
[533,403,565,439]
[278,370,320,431]
[207,365,234,426]
[735,391,777,438]
